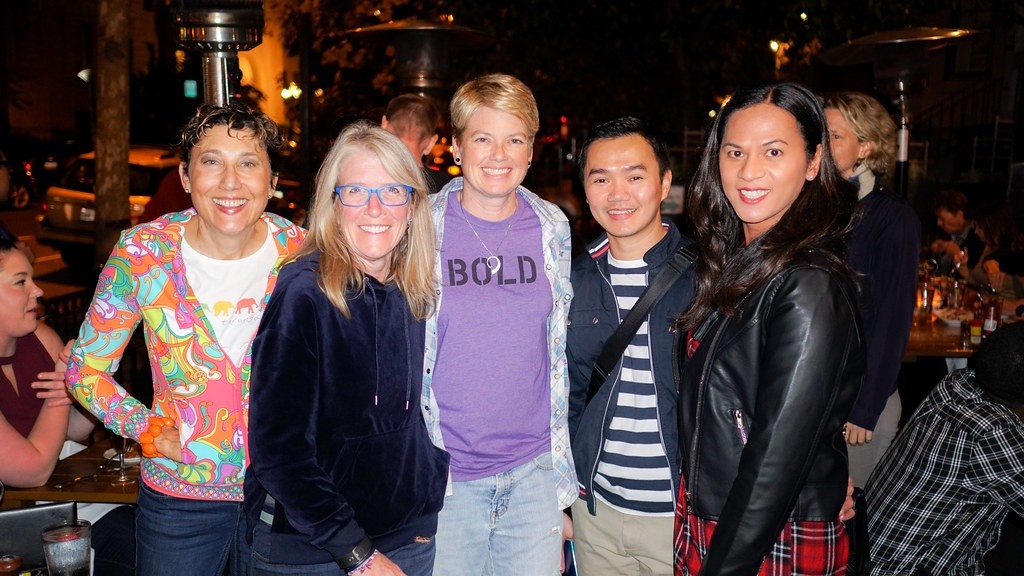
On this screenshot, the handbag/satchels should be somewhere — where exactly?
[844,487,872,576]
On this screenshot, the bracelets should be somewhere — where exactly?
[347,549,378,576]
[138,415,175,458]
[336,538,371,570]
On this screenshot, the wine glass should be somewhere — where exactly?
[110,431,135,486]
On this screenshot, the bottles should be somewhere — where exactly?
[968,312,982,346]
[960,321,970,350]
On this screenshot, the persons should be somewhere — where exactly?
[0,164,35,264]
[421,73,581,576]
[0,245,98,488]
[228,122,448,576]
[63,105,314,576]
[674,82,844,576]
[380,94,439,195]
[821,93,916,489]
[863,323,1024,576]
[954,208,1024,292]
[562,117,855,576]
[930,190,991,279]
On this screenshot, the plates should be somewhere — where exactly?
[104,449,142,465]
[933,309,961,327]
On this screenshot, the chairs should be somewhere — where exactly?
[44,290,87,343]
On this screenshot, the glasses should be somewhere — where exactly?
[333,183,415,207]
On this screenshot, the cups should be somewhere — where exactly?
[914,279,1004,338]
[40,519,91,576]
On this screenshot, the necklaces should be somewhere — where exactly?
[459,189,518,274]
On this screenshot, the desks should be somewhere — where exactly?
[902,287,1024,361]
[23,138,186,319]
[4,408,144,503]
